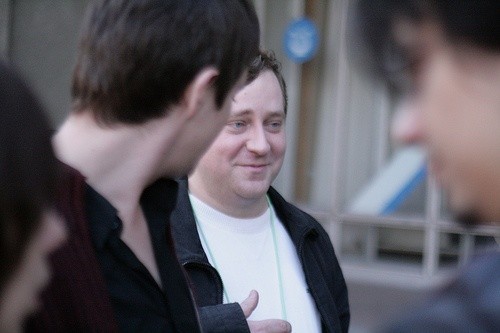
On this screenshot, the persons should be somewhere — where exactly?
[171,50,350,333]
[0,58,67,333]
[345,0,500,333]
[49,0,259,333]
